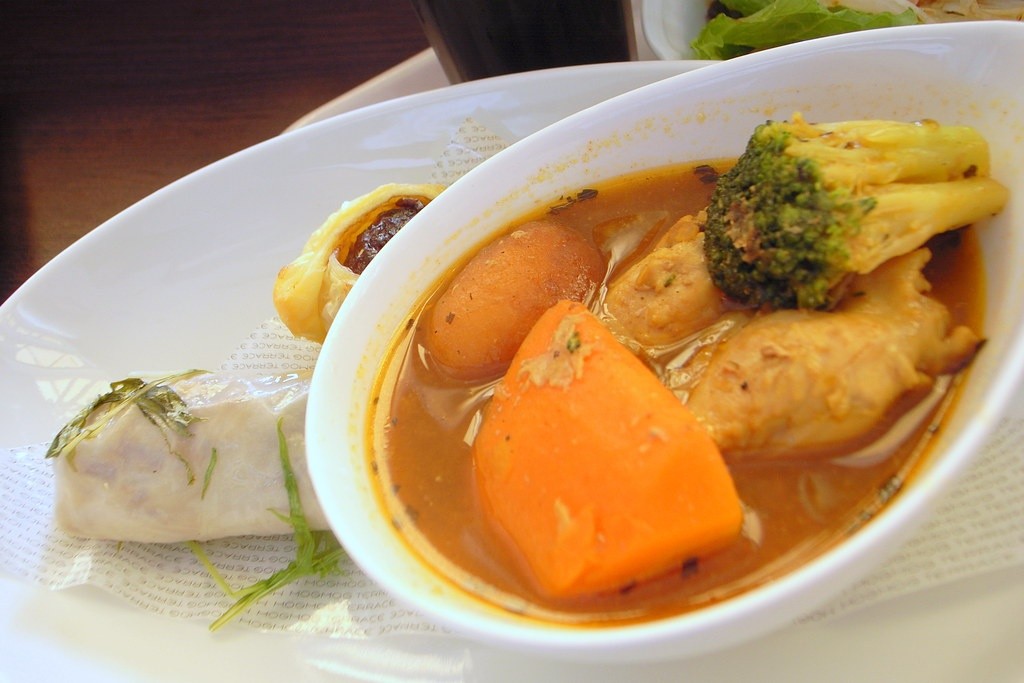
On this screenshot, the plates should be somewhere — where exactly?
[303,24,1024,666]
[1,57,726,683]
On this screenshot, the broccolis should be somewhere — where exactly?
[707,122,1008,309]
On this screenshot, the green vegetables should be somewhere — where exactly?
[688,0,919,61]
[47,371,345,631]
